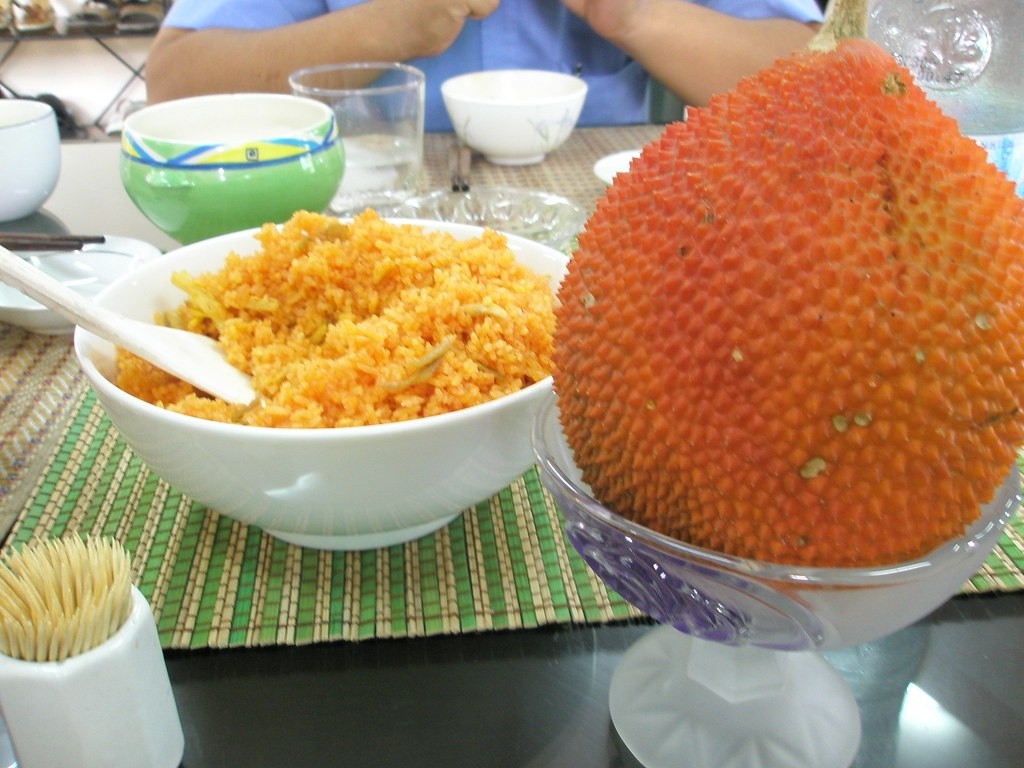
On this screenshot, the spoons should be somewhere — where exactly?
[0,246,257,411]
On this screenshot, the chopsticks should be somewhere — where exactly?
[0,229,107,250]
[450,141,470,193]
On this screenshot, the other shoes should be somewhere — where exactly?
[0,0,10,29]
[12,0,56,32]
[115,0,166,30]
[66,0,123,27]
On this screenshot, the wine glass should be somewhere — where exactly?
[531,397,1020,768]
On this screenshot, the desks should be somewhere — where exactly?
[0,123,1023,768]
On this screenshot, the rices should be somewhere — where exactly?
[107,207,556,431]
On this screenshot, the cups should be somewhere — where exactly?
[290,63,426,217]
[0,585,185,768]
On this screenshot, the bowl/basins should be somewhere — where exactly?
[121,93,345,243]
[0,98,61,222]
[75,221,570,551]
[443,69,588,166]
[0,235,163,334]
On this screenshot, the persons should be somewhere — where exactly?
[143,0,825,132]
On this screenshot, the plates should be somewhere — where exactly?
[593,150,644,186]
[390,187,588,252]
